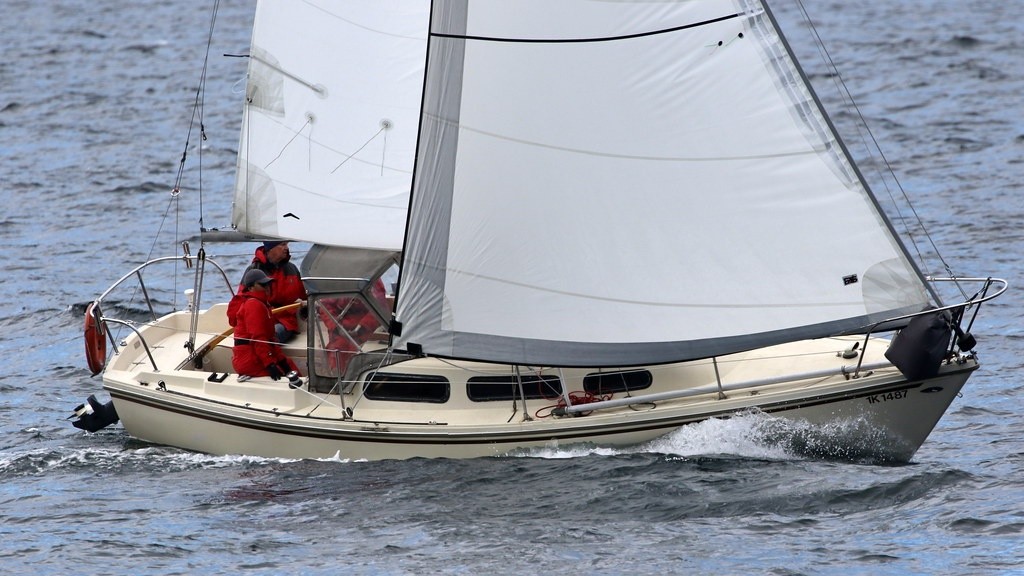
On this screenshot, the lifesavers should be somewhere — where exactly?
[83,301,107,376]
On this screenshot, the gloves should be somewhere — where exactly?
[267,362,283,381]
[299,305,308,319]
[278,359,293,375]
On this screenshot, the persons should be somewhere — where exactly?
[232,269,302,377]
[318,278,385,379]
[238,241,308,345]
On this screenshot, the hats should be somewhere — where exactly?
[244,269,274,284]
[263,240,284,253]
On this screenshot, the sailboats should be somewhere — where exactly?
[64,1,1011,465]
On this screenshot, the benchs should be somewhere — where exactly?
[216,331,329,376]
[175,369,309,409]
